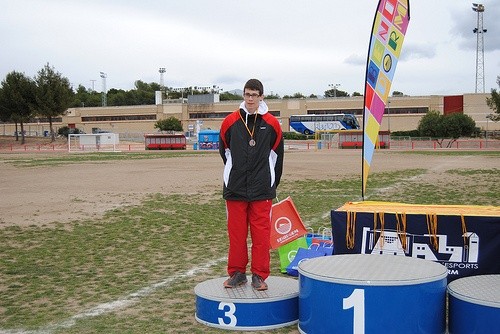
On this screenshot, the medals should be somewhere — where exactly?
[249,139,256,146]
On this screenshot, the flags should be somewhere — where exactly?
[362,0,410,198]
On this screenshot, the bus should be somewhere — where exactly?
[143,134,187,150]
[289,113,360,136]
[197,130,222,150]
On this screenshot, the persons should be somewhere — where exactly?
[219,80,284,290]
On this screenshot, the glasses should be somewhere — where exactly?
[243,94,261,99]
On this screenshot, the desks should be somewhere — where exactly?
[330,201,500,284]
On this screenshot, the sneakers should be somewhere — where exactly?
[251,274,268,290]
[224,271,247,288]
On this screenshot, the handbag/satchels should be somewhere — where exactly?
[277,226,334,276]
[270,196,308,250]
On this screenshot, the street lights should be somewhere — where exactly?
[159,67,166,86]
[90,79,96,90]
[330,84,341,97]
[100,72,108,107]
[472,2,487,93]
[387,100,391,132]
